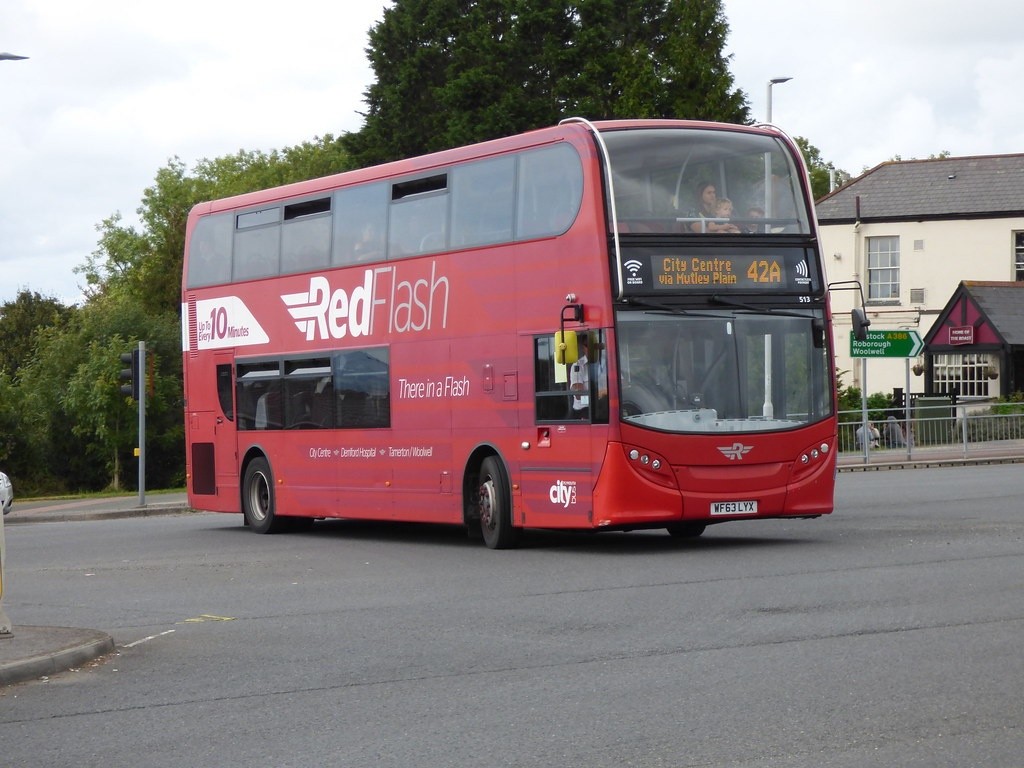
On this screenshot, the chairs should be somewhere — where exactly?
[221,388,327,428]
[420,233,441,251]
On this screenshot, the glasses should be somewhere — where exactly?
[582,343,594,348]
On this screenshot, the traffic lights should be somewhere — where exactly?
[118,349,138,401]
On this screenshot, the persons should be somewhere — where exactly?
[686,182,741,233]
[353,220,380,254]
[856,422,879,450]
[569,335,607,418]
[883,416,905,448]
[743,207,765,233]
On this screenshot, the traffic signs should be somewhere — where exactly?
[850,330,924,357]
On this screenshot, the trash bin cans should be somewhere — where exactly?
[914,397,952,442]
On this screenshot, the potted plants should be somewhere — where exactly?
[983,361,999,380]
[913,356,925,376]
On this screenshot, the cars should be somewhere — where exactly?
[0,472,14,516]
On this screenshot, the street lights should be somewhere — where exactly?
[760,76,794,420]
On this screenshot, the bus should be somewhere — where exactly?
[180,115,871,550]
